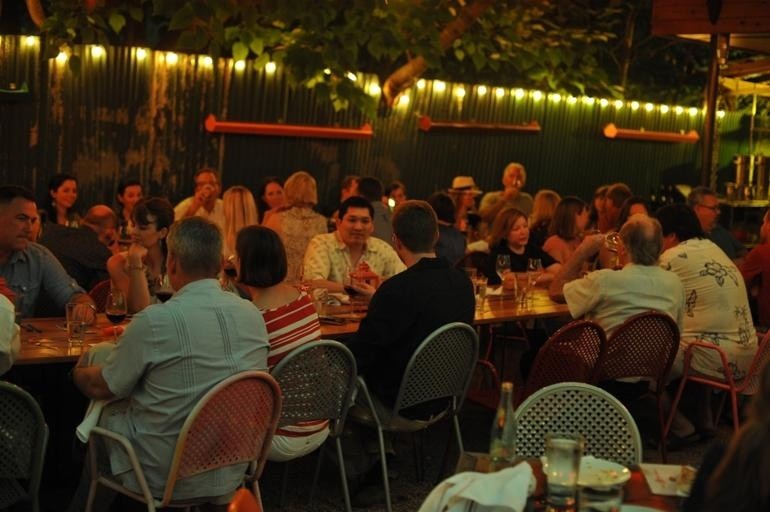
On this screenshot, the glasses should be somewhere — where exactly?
[698,204,718,210]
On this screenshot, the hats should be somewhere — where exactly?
[446,175,483,196]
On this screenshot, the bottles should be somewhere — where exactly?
[487,381,518,471]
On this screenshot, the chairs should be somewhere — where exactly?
[84,365,273,508]
[308,316,477,511]
[1,378,52,511]
[491,382,646,465]
[434,315,609,492]
[592,306,670,451]
[269,336,361,510]
[661,325,770,450]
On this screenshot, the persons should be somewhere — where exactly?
[658,203,758,449]
[4,188,93,484]
[230,225,322,497]
[73,217,271,512]
[705,359,770,512]
[339,199,476,505]
[1,162,770,401]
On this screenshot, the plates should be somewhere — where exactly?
[541,458,626,492]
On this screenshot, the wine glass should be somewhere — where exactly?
[526,258,545,300]
[342,268,362,318]
[220,261,236,291]
[104,288,129,342]
[197,185,215,204]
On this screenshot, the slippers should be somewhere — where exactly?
[665,424,701,451]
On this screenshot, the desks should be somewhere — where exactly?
[444,449,699,511]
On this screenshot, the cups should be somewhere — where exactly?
[542,430,581,506]
[9,286,25,323]
[471,275,489,313]
[64,301,86,344]
[514,272,533,304]
[495,253,511,282]
[154,273,173,303]
[68,213,79,229]
[603,232,620,251]
[724,183,767,201]
[512,177,521,188]
